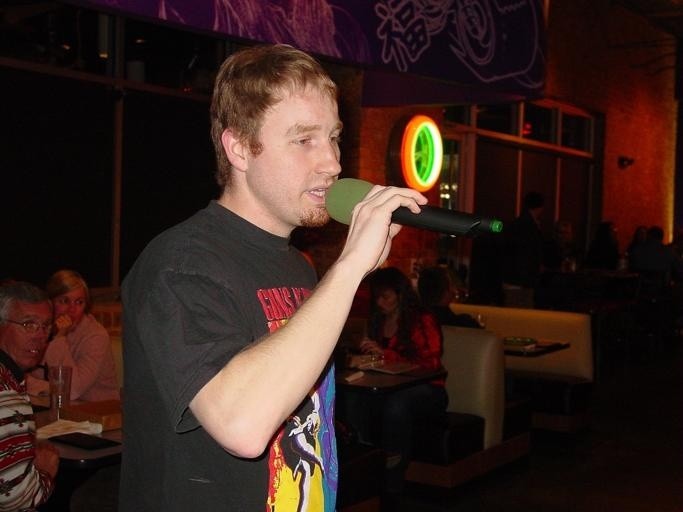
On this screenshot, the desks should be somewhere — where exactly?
[28,408,123,512]
[337,367,449,506]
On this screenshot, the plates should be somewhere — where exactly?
[366,360,421,374]
[501,335,536,345]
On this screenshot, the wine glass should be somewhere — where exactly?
[49,365,72,420]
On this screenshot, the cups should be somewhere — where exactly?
[358,343,385,364]
[473,311,487,328]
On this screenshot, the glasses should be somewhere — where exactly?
[2,318,54,334]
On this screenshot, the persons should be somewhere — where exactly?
[0,280,58,512]
[416,269,482,332]
[44,268,120,401]
[486,191,683,332]
[118,44,427,512]
[360,262,443,456]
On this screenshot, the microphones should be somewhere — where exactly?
[325,177,505,240]
